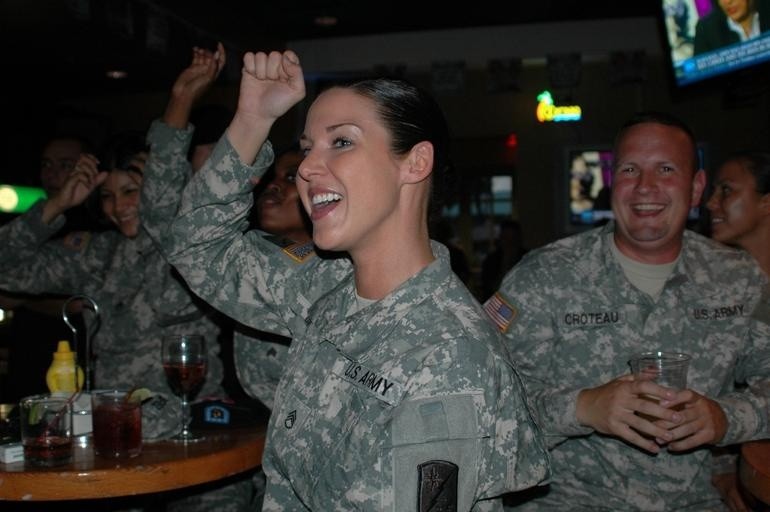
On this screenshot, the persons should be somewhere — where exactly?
[693,0,770,55]
[159,48,552,511]
[481,216,530,300]
[135,40,334,510]
[0,145,226,417]
[478,118,769,512]
[707,144,770,512]
[0,126,106,439]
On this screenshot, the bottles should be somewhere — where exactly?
[45,341,83,399]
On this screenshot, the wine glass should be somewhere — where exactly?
[161,333,209,442]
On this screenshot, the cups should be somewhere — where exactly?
[23,396,74,458]
[628,351,693,422]
[91,390,142,456]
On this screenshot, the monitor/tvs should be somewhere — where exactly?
[653,0,770,103]
[564,148,617,234]
